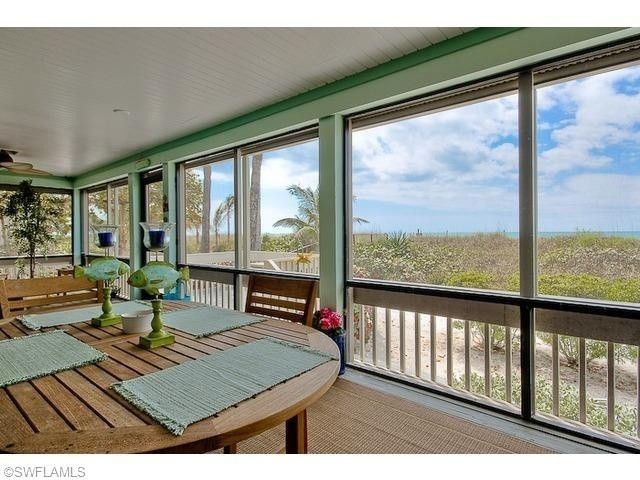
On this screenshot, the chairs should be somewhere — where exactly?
[0,269,320,453]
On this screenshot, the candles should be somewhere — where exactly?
[98,232,113,246]
[148,230,165,248]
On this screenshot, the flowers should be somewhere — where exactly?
[312,307,345,339]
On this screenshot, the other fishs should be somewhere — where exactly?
[127,261,190,296]
[73,256,131,283]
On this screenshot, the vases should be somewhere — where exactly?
[332,335,346,377]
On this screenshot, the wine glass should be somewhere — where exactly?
[139,221,175,263]
[92,225,116,260]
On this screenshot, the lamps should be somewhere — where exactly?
[0,147,51,177]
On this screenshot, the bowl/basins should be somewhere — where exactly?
[120,310,157,334]
